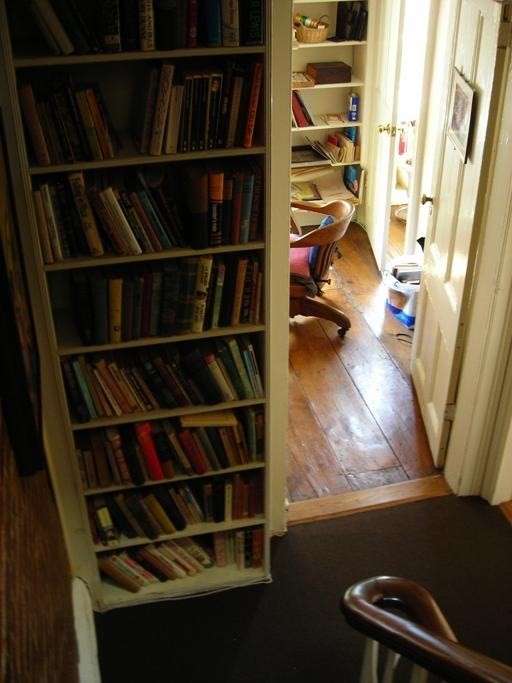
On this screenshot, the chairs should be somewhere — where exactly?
[289,197,356,340]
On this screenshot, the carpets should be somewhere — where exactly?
[92,493,512,683]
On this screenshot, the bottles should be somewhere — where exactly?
[293,12,322,29]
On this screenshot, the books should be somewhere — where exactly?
[7,2,268,166]
[33,160,268,344]
[60,336,267,488]
[88,468,267,592]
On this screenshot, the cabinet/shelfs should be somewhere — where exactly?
[0,0,273,614]
[291,1,374,220]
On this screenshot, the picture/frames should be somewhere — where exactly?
[444,66,477,165]
